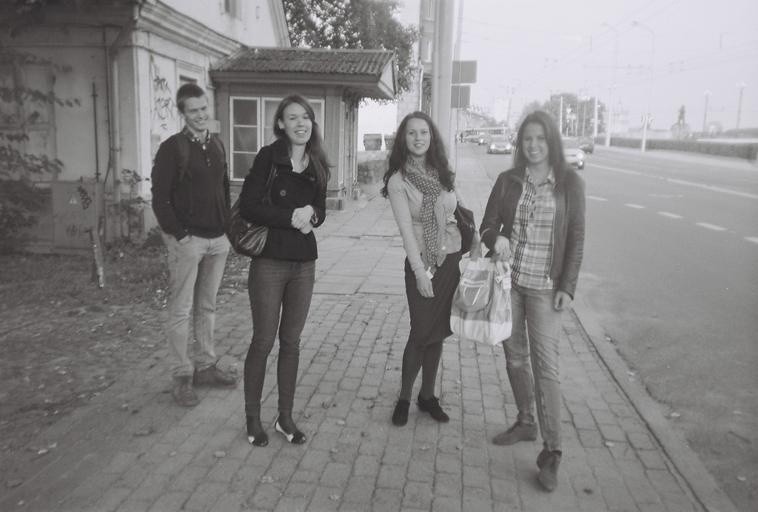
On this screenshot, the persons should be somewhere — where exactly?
[379,110,483,427]
[480,110,587,492]
[149,83,238,407]
[239,94,329,448]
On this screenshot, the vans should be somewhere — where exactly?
[360,127,397,152]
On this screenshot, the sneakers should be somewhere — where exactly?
[537,444,562,490]
[392,398,410,426]
[492,422,537,446]
[172,375,199,406]
[193,364,237,389]
[417,393,450,423]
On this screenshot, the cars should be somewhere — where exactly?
[459,135,511,155]
[576,136,594,154]
[559,136,586,171]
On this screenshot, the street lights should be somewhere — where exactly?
[558,73,658,151]
[701,90,712,132]
[737,81,746,131]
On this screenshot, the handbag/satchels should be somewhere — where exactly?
[449,248,514,346]
[456,202,475,254]
[222,192,273,258]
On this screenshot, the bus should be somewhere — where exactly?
[462,127,512,144]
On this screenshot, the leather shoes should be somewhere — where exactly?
[275,420,306,443]
[247,418,268,447]
